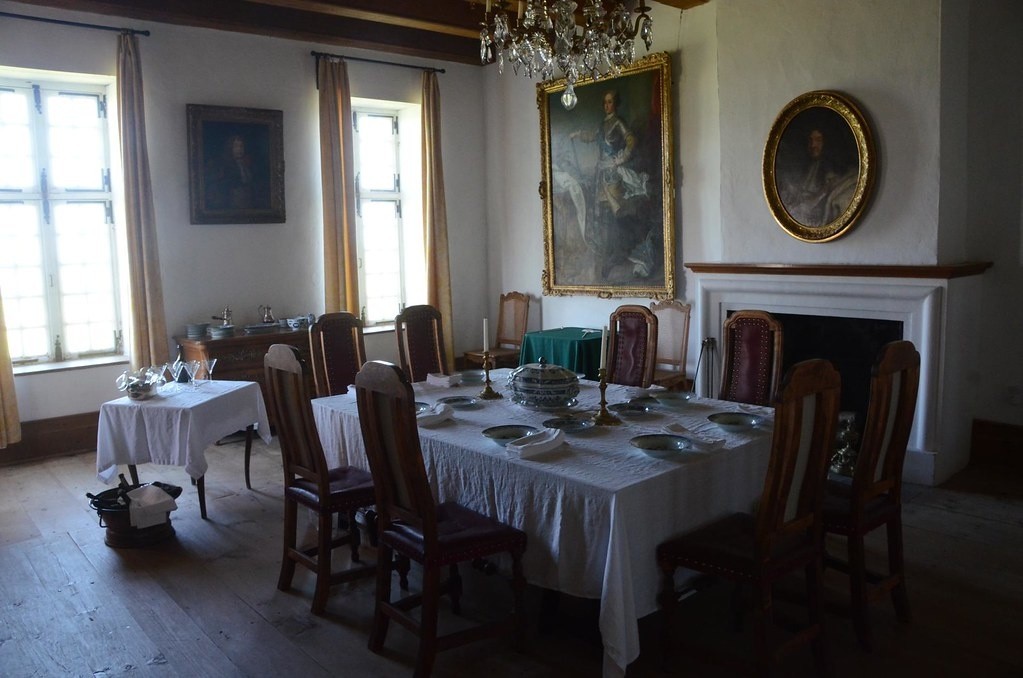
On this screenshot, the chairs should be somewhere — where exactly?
[736,341,921,652]
[717,309,782,409]
[261,345,375,615]
[465,290,531,370]
[653,363,845,678]
[308,312,367,396]
[642,301,694,392]
[600,307,659,389]
[394,305,450,382]
[353,361,526,677]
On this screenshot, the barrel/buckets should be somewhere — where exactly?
[88,484,181,548]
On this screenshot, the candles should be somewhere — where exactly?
[483,318,489,351]
[599,325,608,368]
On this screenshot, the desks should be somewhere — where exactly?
[97,379,272,518]
[174,324,318,443]
[519,327,610,383]
[311,368,855,678]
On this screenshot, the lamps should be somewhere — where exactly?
[481,0,662,109]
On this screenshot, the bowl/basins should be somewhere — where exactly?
[279,317,308,330]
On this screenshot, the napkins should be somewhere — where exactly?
[416,401,458,426]
[661,420,728,455]
[623,384,666,399]
[426,372,463,389]
[505,427,564,460]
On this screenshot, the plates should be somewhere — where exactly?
[186,323,235,338]
[607,402,653,416]
[481,424,540,443]
[707,412,764,431]
[437,395,477,408]
[459,370,485,382]
[629,434,693,457]
[542,417,596,433]
[514,397,578,411]
[650,390,698,405]
[413,401,431,415]
[243,323,279,333]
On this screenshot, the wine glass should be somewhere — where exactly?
[153,358,218,393]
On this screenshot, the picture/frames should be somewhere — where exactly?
[187,103,286,226]
[761,93,877,241]
[537,52,680,299]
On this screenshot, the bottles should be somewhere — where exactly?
[86,474,133,509]
